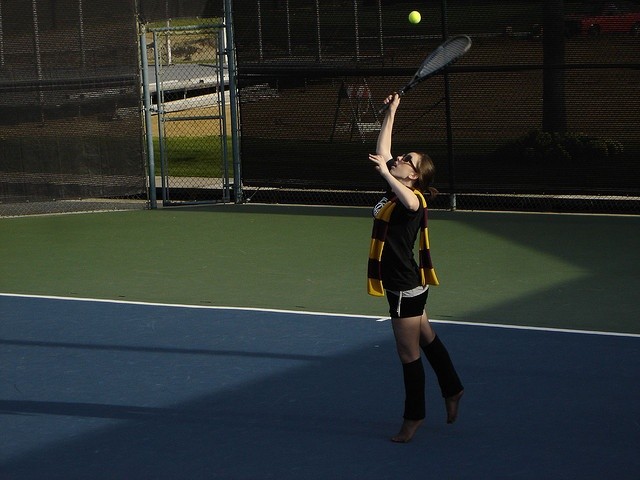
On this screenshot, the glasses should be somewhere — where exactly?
[402,154,417,174]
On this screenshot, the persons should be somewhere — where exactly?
[367,90,465,443]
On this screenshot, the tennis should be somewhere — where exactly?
[408,11,422,25]
